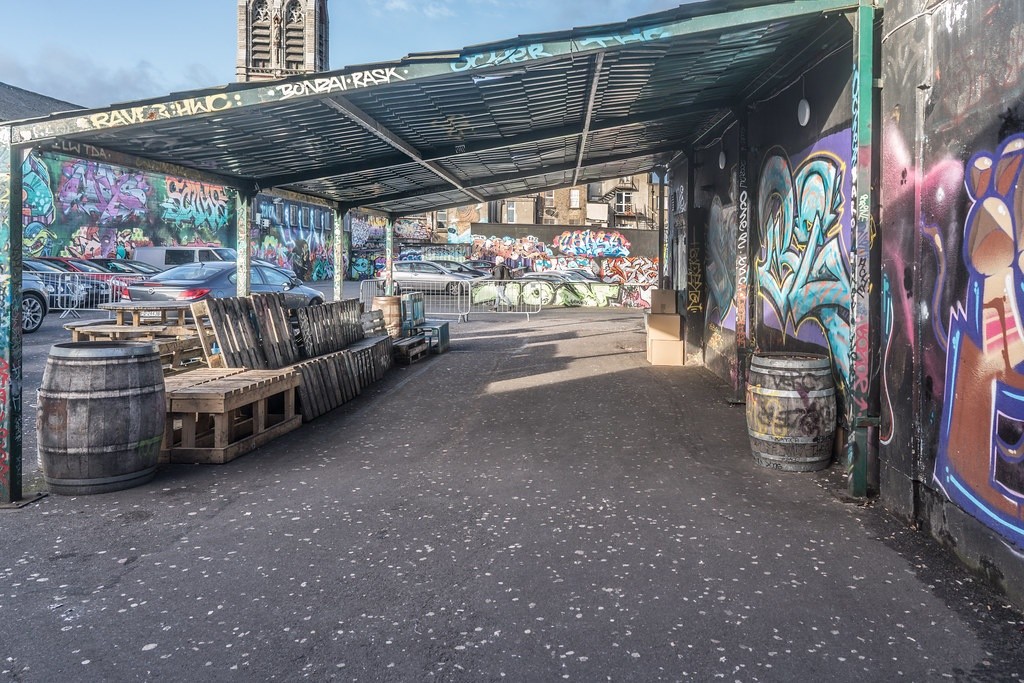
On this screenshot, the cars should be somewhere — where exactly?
[376,260,474,296]
[514,267,602,285]
[19,259,164,334]
[428,260,494,281]
[463,259,496,273]
[119,261,326,340]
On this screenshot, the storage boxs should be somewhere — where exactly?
[646,337,683,366]
[647,313,680,340]
[651,290,676,314]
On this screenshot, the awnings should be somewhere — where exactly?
[0,0,885,511]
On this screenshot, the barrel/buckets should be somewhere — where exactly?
[370,296,402,339]
[35,339,167,495]
[745,351,837,472]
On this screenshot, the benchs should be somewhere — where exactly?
[401,291,449,353]
[391,334,429,364]
[64,301,391,462]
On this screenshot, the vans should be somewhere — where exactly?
[133,246,259,275]
